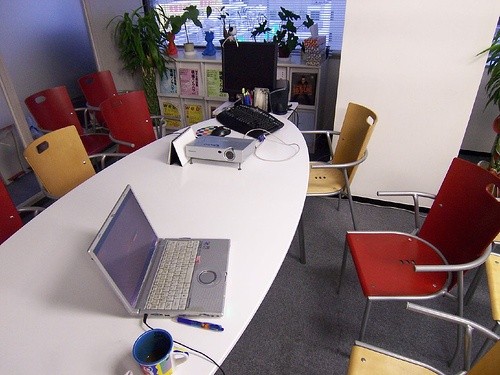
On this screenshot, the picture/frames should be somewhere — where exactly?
[153,59,321,155]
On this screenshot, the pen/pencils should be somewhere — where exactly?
[177,316,224,332]
[241,87,253,106]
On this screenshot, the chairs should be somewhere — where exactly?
[0,70,157,245]
[296,103,500,375]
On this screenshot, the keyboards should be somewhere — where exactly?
[215,104,284,138]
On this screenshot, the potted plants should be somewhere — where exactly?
[477,31,500,170]
[169,6,201,51]
[109,5,169,123]
[272,5,314,59]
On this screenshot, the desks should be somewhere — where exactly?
[0,112,307,375]
[212,102,299,131]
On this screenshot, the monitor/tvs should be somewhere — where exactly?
[222,41,277,101]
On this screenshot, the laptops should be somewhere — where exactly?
[87,184,231,318]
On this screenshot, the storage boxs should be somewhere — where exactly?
[304,36,326,66]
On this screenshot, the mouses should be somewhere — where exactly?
[211,126,231,137]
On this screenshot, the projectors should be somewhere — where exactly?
[185,135,256,164]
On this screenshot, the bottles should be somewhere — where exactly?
[202,31,216,56]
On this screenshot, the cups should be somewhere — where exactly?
[132,328,189,375]
